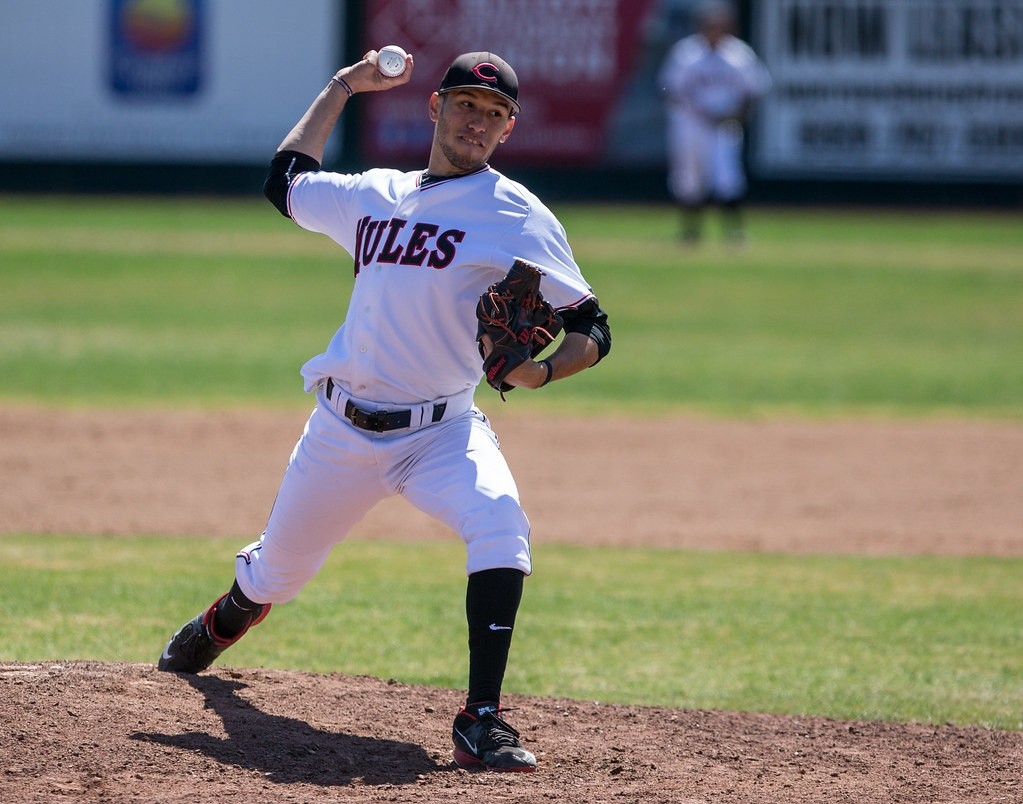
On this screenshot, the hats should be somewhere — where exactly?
[439,52,521,117]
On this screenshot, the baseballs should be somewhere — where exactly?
[376,45,407,78]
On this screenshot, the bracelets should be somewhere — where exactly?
[539,360,552,387]
[333,76,353,96]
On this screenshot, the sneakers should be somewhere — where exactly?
[161,594,271,671]
[451,704,538,772]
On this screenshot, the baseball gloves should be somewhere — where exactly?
[475,260,564,392]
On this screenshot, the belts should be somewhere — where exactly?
[326,378,448,433]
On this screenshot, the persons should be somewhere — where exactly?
[662,14,772,248]
[158,50,612,771]
